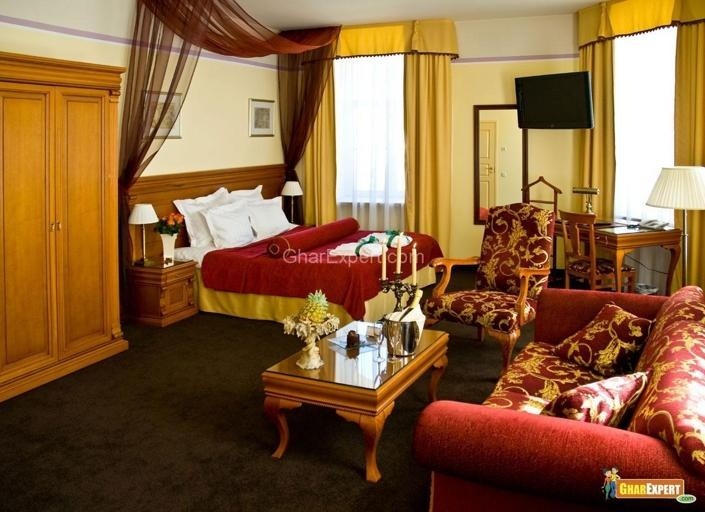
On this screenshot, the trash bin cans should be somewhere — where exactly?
[635,284,659,296]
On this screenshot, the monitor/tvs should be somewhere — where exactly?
[514,71,595,129]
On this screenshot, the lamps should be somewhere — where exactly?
[281,181,304,222]
[573,187,600,214]
[128,204,160,266]
[646,166,705,288]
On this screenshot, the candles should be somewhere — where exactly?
[411,243,417,286]
[381,238,386,279]
[396,232,401,272]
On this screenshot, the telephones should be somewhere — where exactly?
[639,218,671,231]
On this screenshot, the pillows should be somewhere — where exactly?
[540,369,653,426]
[550,301,655,379]
[173,185,360,259]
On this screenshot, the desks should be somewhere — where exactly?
[555,218,681,295]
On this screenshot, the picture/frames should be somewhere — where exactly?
[249,98,275,137]
[139,90,183,139]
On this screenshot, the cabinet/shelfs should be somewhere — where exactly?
[0,51,128,405]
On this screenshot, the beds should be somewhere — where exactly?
[119,164,444,332]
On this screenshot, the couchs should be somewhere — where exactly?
[412,286,704,512]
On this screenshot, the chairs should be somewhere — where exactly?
[559,209,635,292]
[423,203,556,377]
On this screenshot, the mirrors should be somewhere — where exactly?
[474,104,528,225]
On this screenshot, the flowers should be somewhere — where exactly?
[154,213,185,236]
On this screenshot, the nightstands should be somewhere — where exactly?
[126,257,199,329]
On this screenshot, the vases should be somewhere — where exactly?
[160,234,178,267]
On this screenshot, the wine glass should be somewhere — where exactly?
[373,321,403,365]
